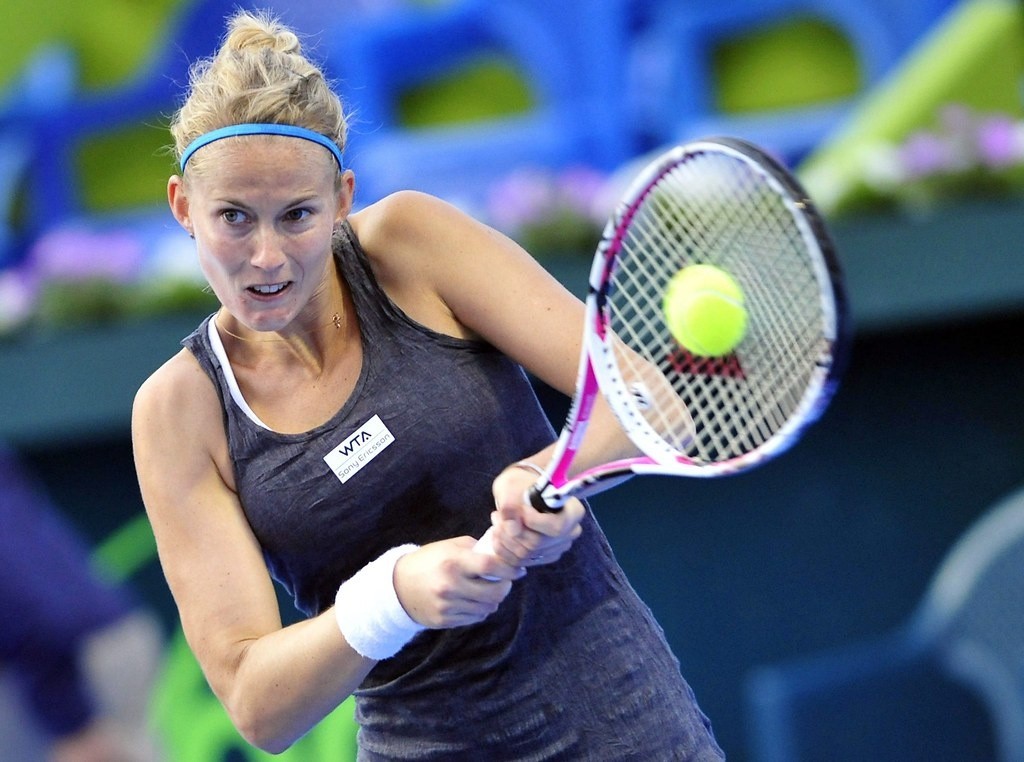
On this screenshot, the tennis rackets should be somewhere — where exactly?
[467,135,854,582]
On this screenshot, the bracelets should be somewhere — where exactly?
[513,462,545,476]
[333,543,428,662]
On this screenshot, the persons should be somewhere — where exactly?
[132,10,734,762]
[0,482,156,760]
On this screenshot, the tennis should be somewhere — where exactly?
[663,263,748,360]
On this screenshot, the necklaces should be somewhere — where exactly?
[214,293,343,343]
